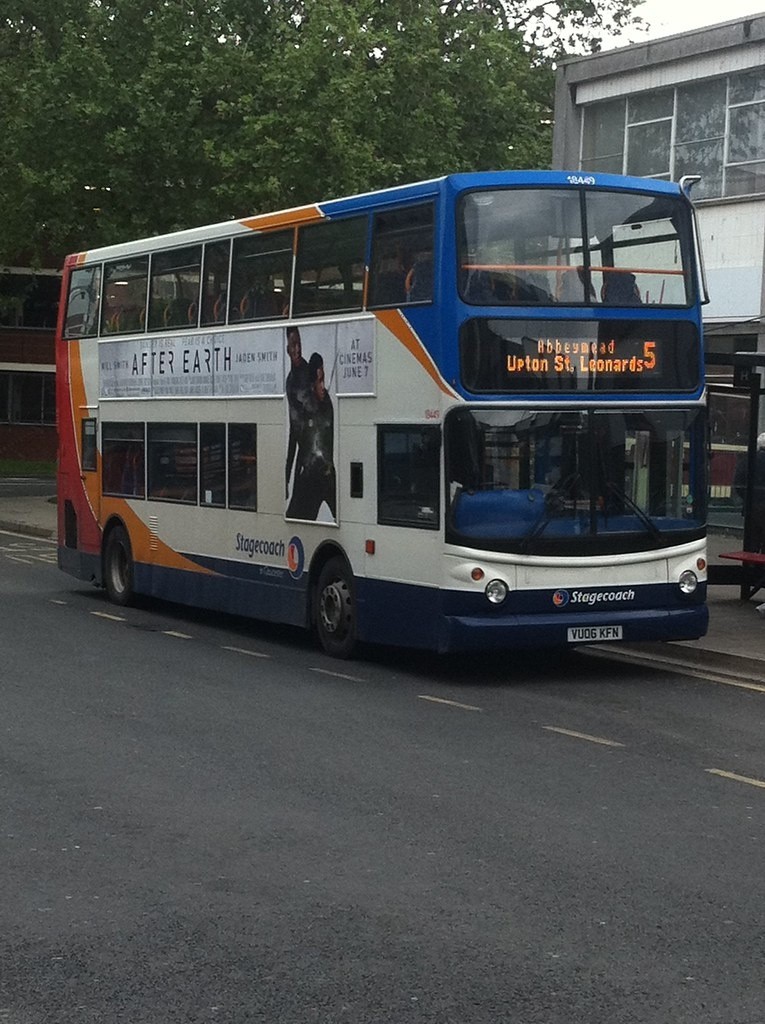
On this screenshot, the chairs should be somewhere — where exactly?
[106,258,435,331]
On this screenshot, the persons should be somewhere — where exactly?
[733,431,765,555]
[286,325,337,522]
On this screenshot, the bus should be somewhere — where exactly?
[53,170,710,659]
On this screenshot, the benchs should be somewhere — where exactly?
[719,551,765,606]
